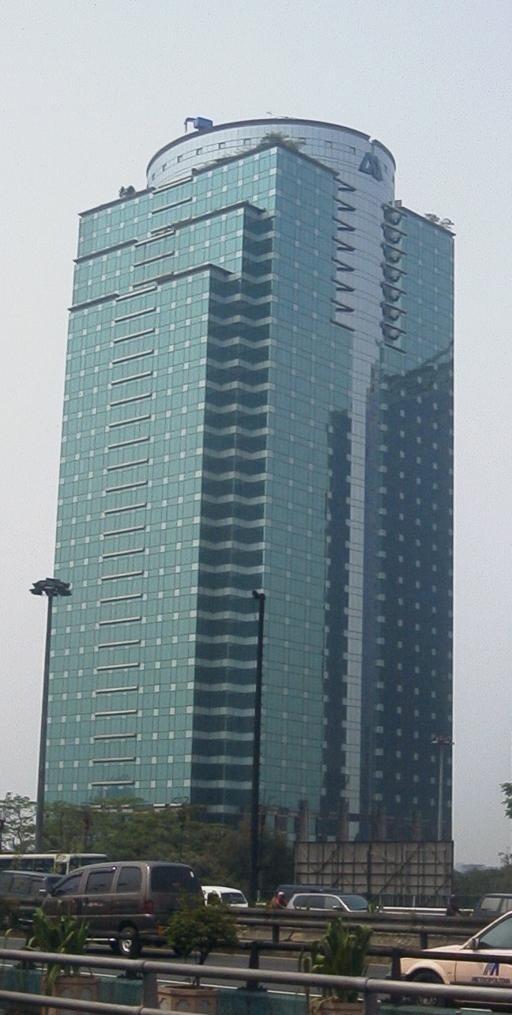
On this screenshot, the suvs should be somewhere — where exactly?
[200,883,248,912]
[473,894,512,923]
[285,892,370,913]
[274,882,340,911]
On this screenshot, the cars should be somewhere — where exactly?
[389,908,512,1012]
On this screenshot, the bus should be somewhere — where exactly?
[1,854,111,879]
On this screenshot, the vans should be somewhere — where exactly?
[0,869,66,929]
[38,860,207,959]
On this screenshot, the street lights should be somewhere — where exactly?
[29,572,74,856]
[236,585,269,992]
[429,736,454,841]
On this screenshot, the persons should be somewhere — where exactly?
[446,894,461,918]
[270,891,289,910]
